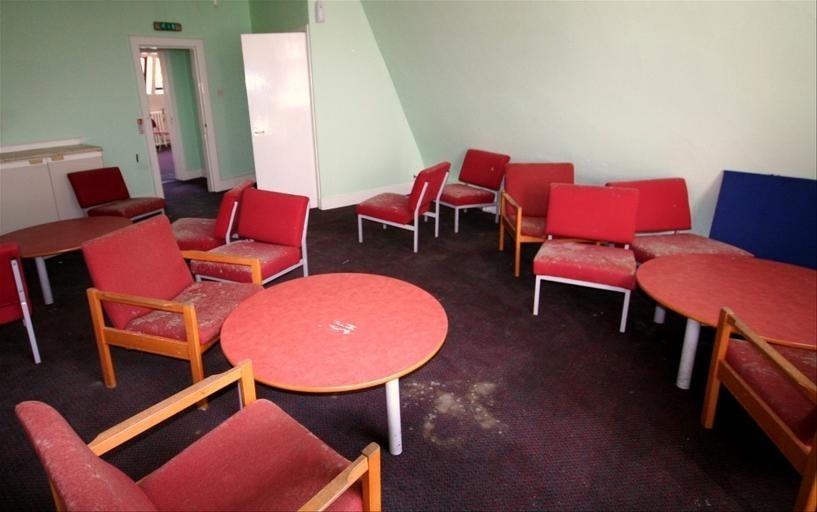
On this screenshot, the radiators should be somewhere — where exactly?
[150,110,169,151]
